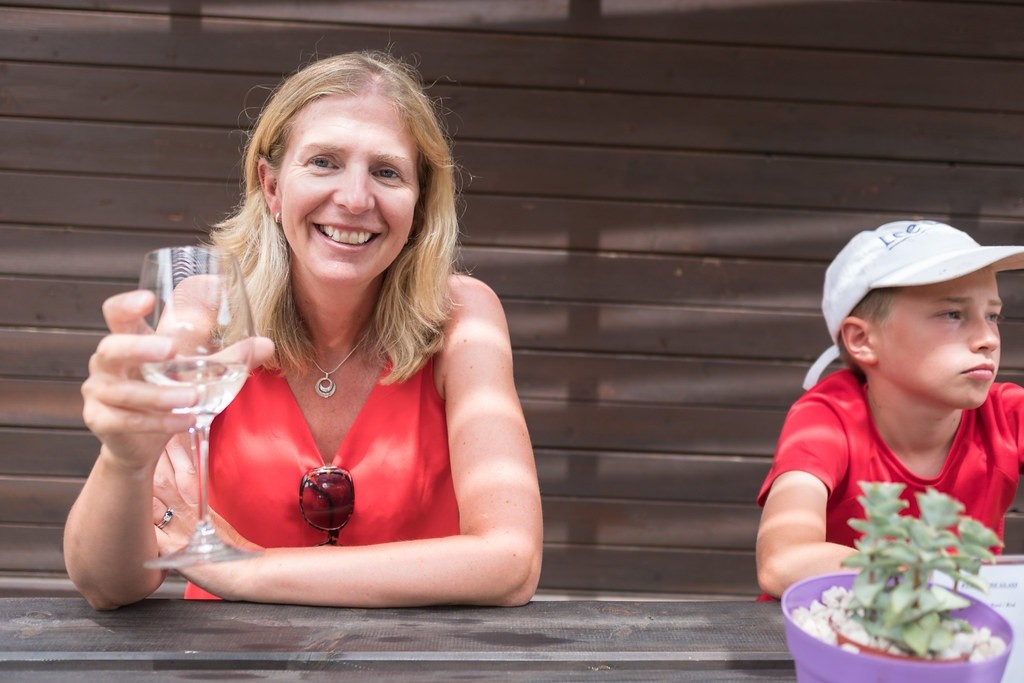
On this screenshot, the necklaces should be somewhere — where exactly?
[310,335,366,398]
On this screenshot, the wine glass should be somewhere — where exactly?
[134,245,266,570]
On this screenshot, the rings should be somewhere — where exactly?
[156,507,173,528]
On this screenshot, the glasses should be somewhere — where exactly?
[299,465,355,547]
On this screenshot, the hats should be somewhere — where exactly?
[802,220,1024,392]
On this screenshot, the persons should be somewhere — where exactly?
[62,49,545,612]
[753,220,1024,602]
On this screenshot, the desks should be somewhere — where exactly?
[0,598,798,683]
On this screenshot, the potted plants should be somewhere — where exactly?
[780,479,1014,683]
